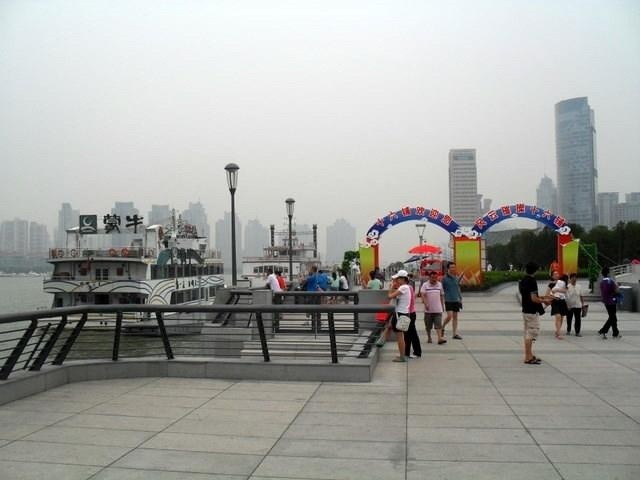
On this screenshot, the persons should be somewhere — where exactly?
[262,258,464,364]
[623,257,631,264]
[543,275,569,311]
[549,259,562,277]
[545,270,568,341]
[632,257,640,264]
[518,262,554,365]
[596,265,623,340]
[565,273,584,337]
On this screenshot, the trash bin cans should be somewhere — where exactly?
[618,286,633,311]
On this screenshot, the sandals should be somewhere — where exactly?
[524,356,542,364]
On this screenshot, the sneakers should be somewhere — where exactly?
[612,334,623,338]
[597,331,607,340]
[438,339,447,344]
[452,334,462,339]
[392,357,407,362]
[574,333,582,337]
[409,353,418,358]
[441,328,444,337]
[566,331,570,335]
[427,338,433,343]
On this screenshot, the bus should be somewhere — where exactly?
[420,259,449,287]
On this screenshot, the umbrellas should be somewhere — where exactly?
[408,241,442,258]
[403,255,429,263]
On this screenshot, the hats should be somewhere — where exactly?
[391,270,408,279]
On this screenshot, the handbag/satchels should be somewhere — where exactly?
[610,290,624,305]
[395,315,411,331]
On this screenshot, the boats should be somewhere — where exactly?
[238,225,321,289]
[36,209,225,331]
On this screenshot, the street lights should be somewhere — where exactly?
[285,198,297,280]
[224,162,240,286]
[416,223,426,246]
